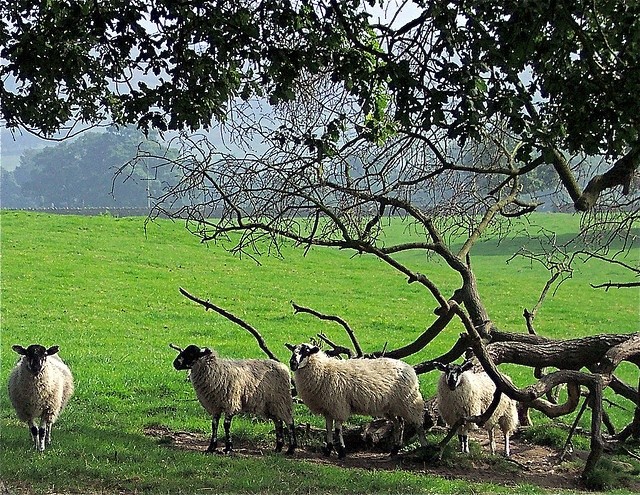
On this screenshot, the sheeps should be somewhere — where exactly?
[8,344,75,455]
[433,360,521,456]
[284,341,435,460]
[168,342,297,456]
